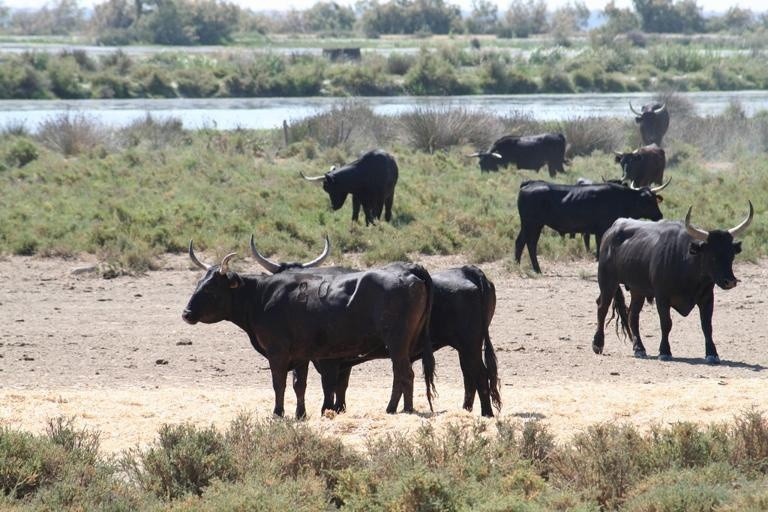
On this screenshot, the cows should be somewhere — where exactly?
[465,132,570,177]
[630,100,670,147]
[591,200,754,364]
[182,240,438,419]
[514,176,672,279]
[299,149,398,227]
[250,233,502,418]
[613,142,665,189]
